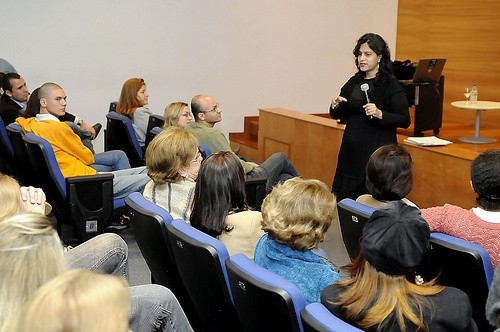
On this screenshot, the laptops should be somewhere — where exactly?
[399,59,447,83]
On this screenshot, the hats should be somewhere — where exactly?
[362,201,430,274]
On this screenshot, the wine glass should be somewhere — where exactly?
[463,87,471,106]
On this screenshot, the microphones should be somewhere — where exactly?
[361,83,374,119]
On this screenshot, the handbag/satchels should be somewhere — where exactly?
[393,59,418,107]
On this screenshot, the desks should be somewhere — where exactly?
[450,101,500,144]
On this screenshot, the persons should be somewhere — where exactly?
[189,151,266,262]
[143,127,203,224]
[0,72,102,153]
[16,82,150,201]
[187,95,299,192]
[356,144,419,209]
[24,88,130,171]
[163,102,192,127]
[23,269,133,332]
[421,149,500,270]
[320,201,478,332]
[254,177,342,304]
[329,33,411,260]
[0,212,194,332]
[0,173,130,279]
[116,78,153,146]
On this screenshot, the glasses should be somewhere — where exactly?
[187,153,203,164]
[203,105,219,113]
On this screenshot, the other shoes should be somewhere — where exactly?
[91,123,101,140]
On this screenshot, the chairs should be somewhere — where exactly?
[0,102,495,332]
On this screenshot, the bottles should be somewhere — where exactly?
[471,83,478,103]
